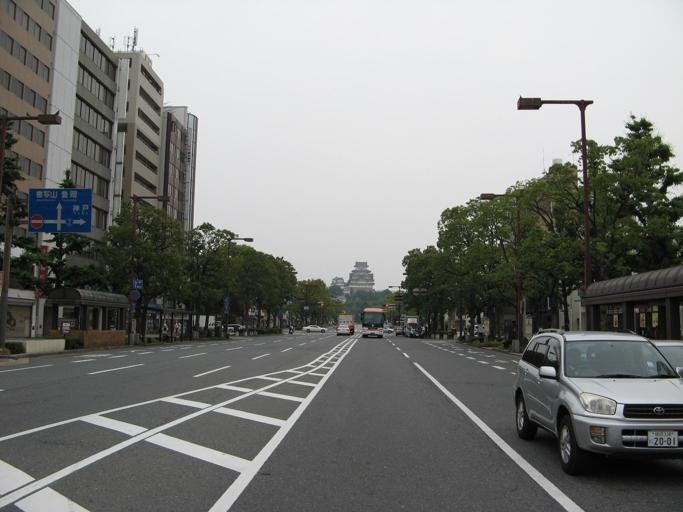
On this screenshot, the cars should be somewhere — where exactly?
[336,323,354,335]
[396,327,404,335]
[303,325,327,333]
[512,328,683,474]
[384,327,394,333]
[208,321,245,336]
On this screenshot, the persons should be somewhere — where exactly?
[162,323,168,343]
[349,320,354,328]
[290,324,293,329]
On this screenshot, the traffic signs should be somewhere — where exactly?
[28,189,92,232]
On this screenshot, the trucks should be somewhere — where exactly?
[406,316,416,334]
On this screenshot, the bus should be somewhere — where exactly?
[361,307,384,338]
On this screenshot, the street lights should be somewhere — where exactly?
[130,194,170,346]
[1,110,61,126]
[518,98,594,288]
[481,193,519,350]
[225,237,253,339]
[387,286,401,325]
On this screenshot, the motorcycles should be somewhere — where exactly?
[288,324,297,333]
[456,325,485,345]
